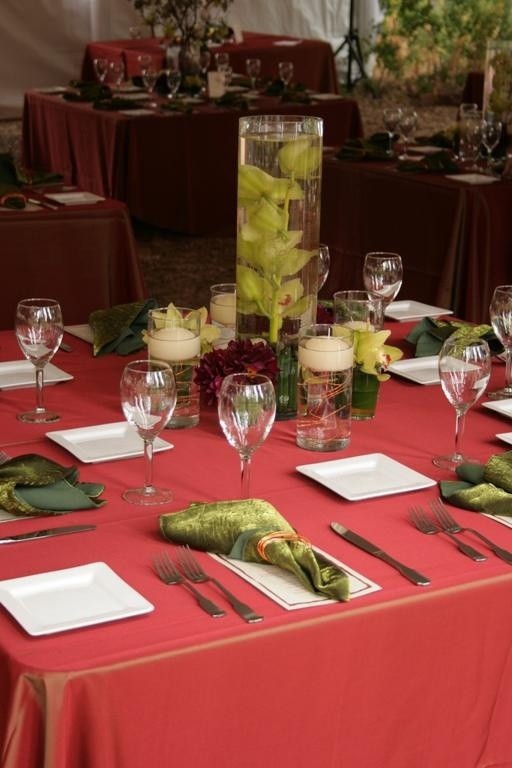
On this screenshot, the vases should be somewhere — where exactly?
[351,369,380,420]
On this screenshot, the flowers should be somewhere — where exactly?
[193,326,281,413]
[230,135,328,342]
[122,0,235,98]
[321,316,404,381]
[361,0,511,110]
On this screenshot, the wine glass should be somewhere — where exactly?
[120,359,176,508]
[17,297,62,422]
[431,335,490,471]
[93,54,294,106]
[381,101,503,172]
[489,286,512,400]
[364,250,404,327]
[217,372,277,499]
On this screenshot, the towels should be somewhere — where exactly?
[60,79,115,102]
[158,492,354,603]
[435,443,512,522]
[0,451,110,517]
[12,164,66,189]
[334,123,472,174]
[397,313,505,367]
[85,295,158,360]
[159,76,319,117]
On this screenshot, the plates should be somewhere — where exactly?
[481,394,512,449]
[43,190,108,207]
[0,559,158,638]
[388,300,453,321]
[45,417,175,465]
[61,323,94,345]
[295,451,438,501]
[388,353,482,386]
[1,360,73,392]
[445,174,500,186]
[36,86,75,95]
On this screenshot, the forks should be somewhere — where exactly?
[427,498,511,567]
[151,550,227,619]
[408,505,488,562]
[172,543,263,623]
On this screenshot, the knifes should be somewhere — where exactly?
[330,521,432,587]
[58,342,74,354]
[28,198,60,211]
[0,524,97,545]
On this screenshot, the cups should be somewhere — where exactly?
[211,284,236,351]
[148,306,202,429]
[294,324,352,449]
[334,291,384,423]
[315,243,331,292]
